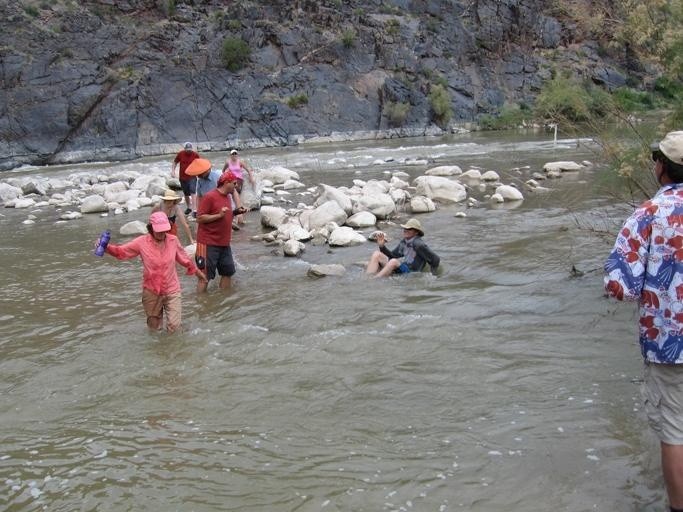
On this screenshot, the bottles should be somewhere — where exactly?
[94,231,112,257]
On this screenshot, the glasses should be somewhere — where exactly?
[232,153,237,155]
[652,150,663,164]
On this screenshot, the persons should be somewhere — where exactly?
[184,159,242,242]
[364,219,440,278]
[171,142,200,218]
[195,171,249,292]
[152,191,194,245]
[603,129,682,512]
[222,150,252,225]
[93,212,208,334]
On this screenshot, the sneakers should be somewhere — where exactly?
[184,209,191,214]
[192,211,196,218]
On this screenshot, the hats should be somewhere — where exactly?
[184,158,210,176]
[148,212,171,232]
[399,218,423,237]
[231,150,237,154]
[159,190,181,205]
[184,142,192,150]
[649,130,682,166]
